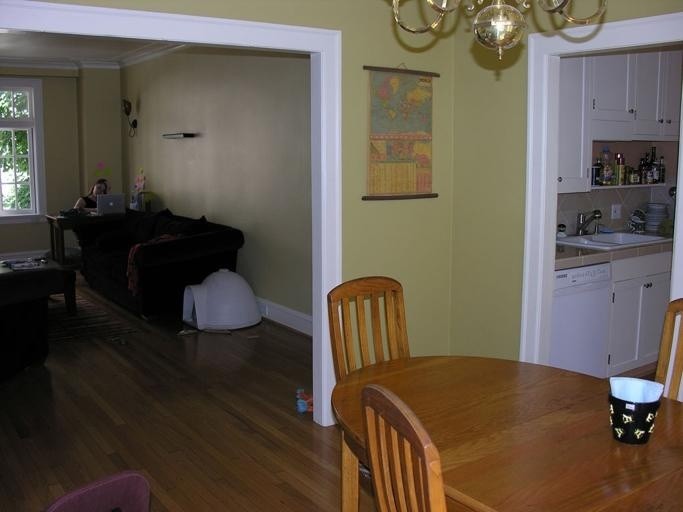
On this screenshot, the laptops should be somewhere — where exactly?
[91,192,126,216]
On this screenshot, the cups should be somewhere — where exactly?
[607,390,664,446]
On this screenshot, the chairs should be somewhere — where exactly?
[361,383,446,512]
[655,297,683,402]
[326,277,410,493]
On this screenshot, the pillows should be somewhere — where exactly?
[125,206,212,238]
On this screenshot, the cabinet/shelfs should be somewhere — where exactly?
[611,251,672,376]
[633,51,682,136]
[591,54,637,121]
[557,56,592,193]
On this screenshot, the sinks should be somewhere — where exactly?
[556,233,673,250]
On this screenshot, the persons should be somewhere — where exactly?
[74,178,112,215]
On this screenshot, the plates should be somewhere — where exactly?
[646,202,668,233]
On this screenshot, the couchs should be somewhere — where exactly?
[78,212,245,323]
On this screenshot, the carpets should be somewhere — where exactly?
[47,292,136,344]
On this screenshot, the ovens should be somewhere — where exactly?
[551,262,614,380]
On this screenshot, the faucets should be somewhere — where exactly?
[577,210,601,234]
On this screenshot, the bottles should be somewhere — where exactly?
[592,147,669,184]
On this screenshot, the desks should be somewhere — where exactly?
[45,210,149,265]
[331,354,682,512]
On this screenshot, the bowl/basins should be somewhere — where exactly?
[629,208,645,233]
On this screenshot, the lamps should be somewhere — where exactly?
[120,98,136,136]
[392,0,607,60]
